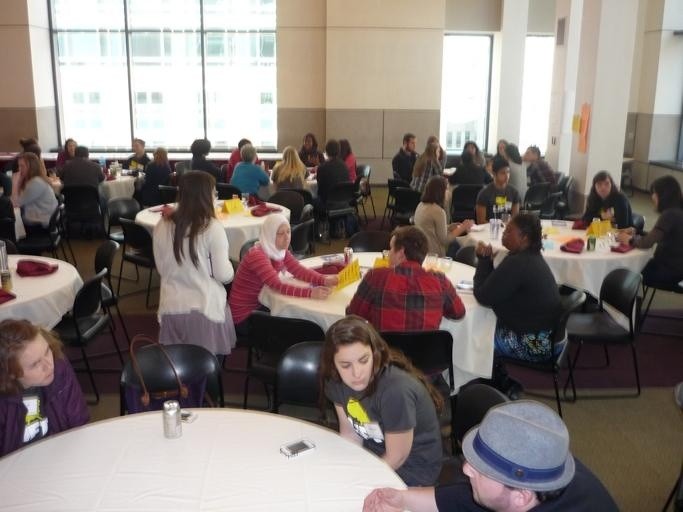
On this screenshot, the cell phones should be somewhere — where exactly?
[181,412,196,424]
[280,440,315,457]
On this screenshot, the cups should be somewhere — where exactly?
[487,217,506,241]
[427,253,453,273]
[214,190,217,200]
[502,212,511,224]
[590,209,618,253]
[108,161,121,178]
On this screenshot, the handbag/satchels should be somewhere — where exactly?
[129,332,214,413]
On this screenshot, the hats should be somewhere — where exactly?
[460,400,575,492]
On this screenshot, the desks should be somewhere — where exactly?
[0,406,409,511]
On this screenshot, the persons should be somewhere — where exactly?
[392,135,558,257]
[474,211,570,401]
[1,318,92,458]
[363,397,623,511]
[151,170,237,356]
[228,213,339,342]
[346,225,466,336]
[316,313,446,489]
[583,170,635,230]
[615,175,683,291]
[184,133,358,257]
[0,133,173,260]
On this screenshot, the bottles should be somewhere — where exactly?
[163,400,183,438]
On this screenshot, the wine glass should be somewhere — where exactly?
[240,191,249,210]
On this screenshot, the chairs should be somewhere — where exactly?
[0,158,683,460]
